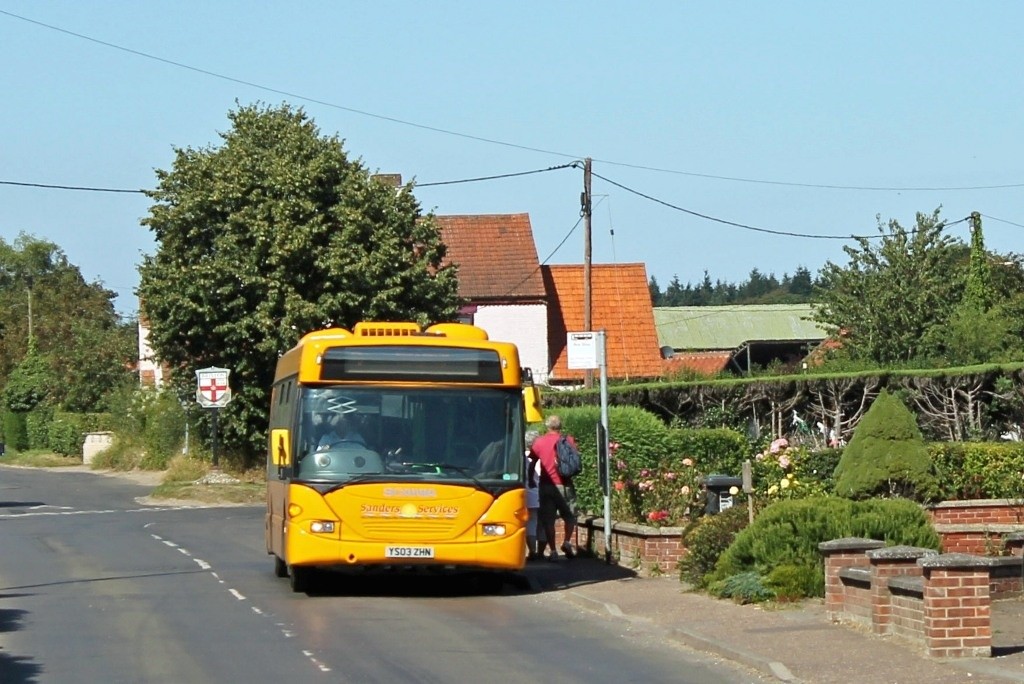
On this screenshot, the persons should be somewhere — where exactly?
[318,418,366,450]
[527,415,578,560]
[522,430,548,561]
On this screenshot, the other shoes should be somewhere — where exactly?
[527,553,536,562]
[549,553,559,561]
[535,553,547,563]
[561,542,574,559]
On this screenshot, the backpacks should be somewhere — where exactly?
[554,435,582,477]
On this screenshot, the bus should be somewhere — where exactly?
[264,322,546,592]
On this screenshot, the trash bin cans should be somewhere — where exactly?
[699,476,743,516]
